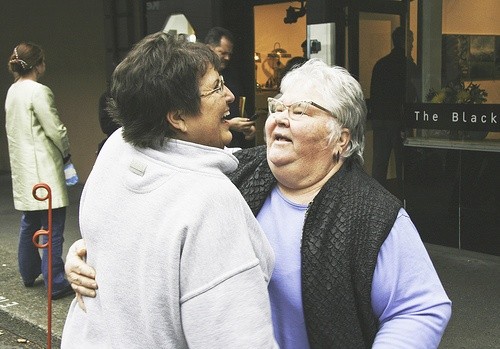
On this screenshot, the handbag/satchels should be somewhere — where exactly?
[63,160,79,187]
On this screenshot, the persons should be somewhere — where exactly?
[368,26,419,200]
[441,37,469,88]
[300,39,307,61]
[64,57,452,349]
[60,29,280,349]
[202,26,258,149]
[4,41,75,299]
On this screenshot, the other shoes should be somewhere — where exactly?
[51,285,75,299]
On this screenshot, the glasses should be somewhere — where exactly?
[267,97,346,124]
[200,75,225,97]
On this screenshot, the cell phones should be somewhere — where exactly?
[247,114,260,122]
